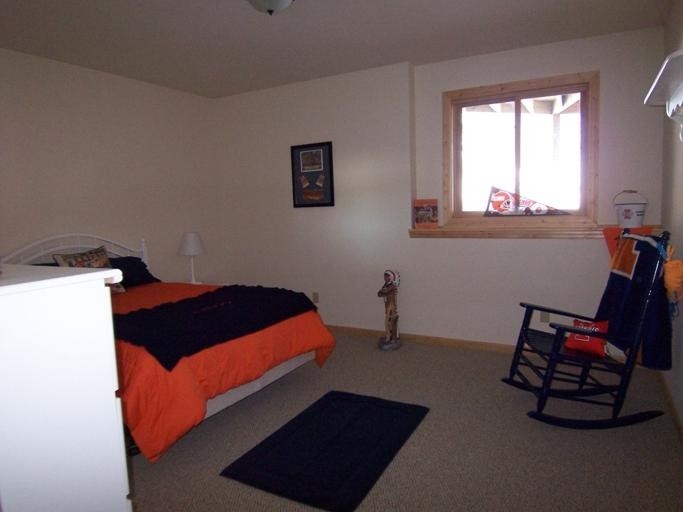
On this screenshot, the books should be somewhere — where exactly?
[414,199,437,229]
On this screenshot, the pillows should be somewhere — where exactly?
[564,319,608,358]
[50,245,125,296]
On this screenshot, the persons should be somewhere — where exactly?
[378,269,399,343]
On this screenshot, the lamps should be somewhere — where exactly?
[175,232,204,284]
[247,0,295,17]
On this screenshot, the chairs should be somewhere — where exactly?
[501,227,670,428]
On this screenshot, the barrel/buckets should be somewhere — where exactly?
[612,191,649,230]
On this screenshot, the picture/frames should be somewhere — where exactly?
[288,139,333,208]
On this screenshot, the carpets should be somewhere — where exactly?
[216,388,429,511]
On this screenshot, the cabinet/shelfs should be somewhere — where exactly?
[0,265,134,512]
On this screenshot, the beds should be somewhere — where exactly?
[0,232,316,459]
